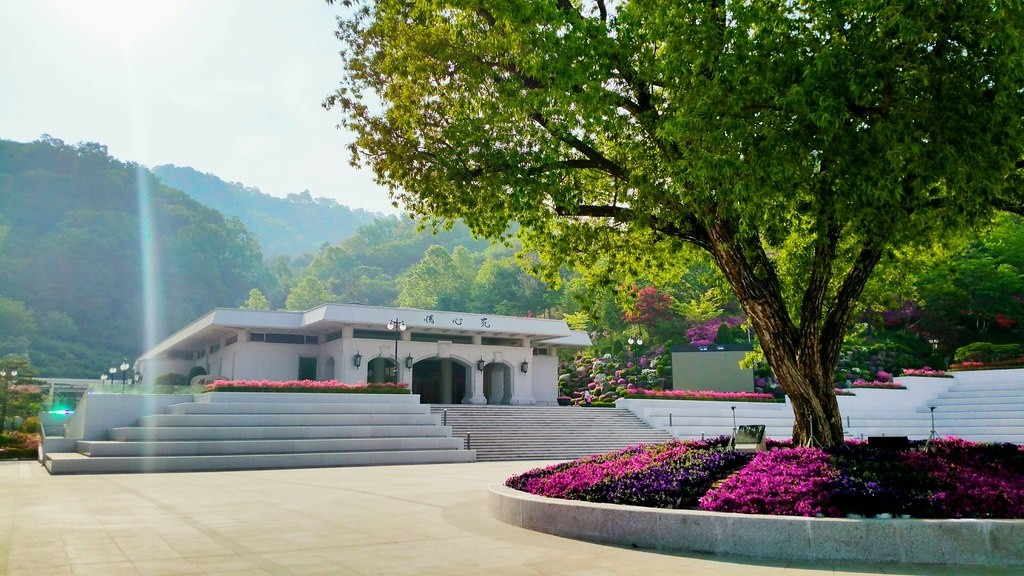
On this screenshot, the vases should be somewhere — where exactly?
[557,399,571,406]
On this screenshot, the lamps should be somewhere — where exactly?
[354,349,363,370]
[405,352,414,371]
[734,424,767,453]
[477,355,485,374]
[521,358,529,375]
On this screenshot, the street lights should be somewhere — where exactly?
[0,371,17,436]
[388,320,407,383]
[102,355,130,392]
[628,332,643,385]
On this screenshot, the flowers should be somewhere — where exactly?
[556,396,571,400]
